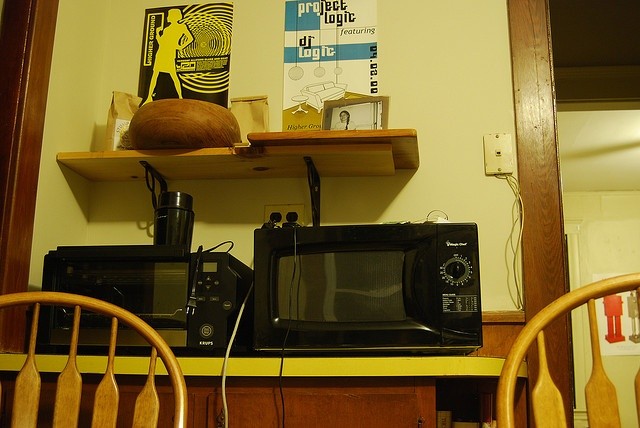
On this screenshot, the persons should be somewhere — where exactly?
[334,110,356,130]
[141,9,195,106]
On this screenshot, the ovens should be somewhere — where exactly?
[38,245,251,357]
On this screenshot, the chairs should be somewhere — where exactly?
[493,268,640,427]
[1,289,187,427]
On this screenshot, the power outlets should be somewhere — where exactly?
[264,204,305,230]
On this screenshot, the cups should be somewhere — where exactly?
[137,159,192,244]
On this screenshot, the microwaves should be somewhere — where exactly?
[252,219,483,356]
